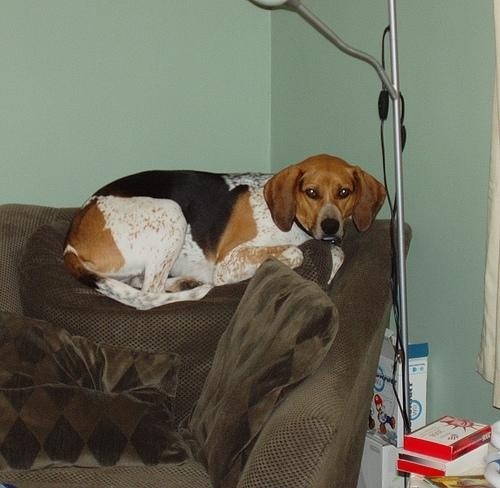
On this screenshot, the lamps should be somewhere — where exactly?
[251,0,413,435]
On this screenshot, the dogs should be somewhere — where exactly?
[61,153,387,312]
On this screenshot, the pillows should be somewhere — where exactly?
[1,310,188,473]
[178,255,340,487]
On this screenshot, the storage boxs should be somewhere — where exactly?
[368,329,429,447]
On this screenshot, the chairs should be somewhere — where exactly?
[1,202,412,488]
